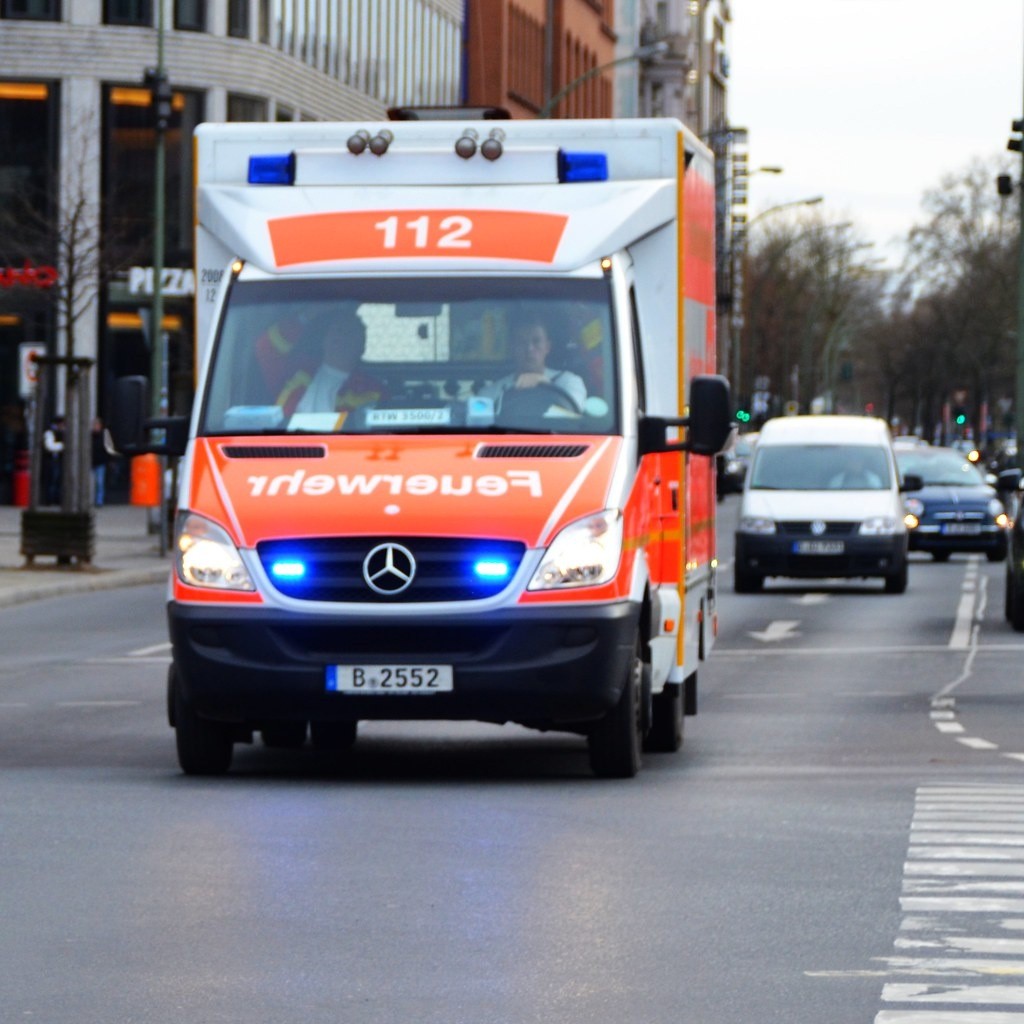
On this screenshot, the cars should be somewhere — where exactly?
[998,469,1024,629]
[891,435,1011,562]
[725,431,759,491]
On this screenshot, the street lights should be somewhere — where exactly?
[540,40,898,417]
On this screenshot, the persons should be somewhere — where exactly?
[42,415,118,508]
[473,314,587,414]
[251,303,389,416]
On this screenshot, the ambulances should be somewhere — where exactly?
[103,116,733,773]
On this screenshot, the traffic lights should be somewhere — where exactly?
[956,406,966,432]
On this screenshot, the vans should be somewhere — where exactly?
[732,416,910,596]
